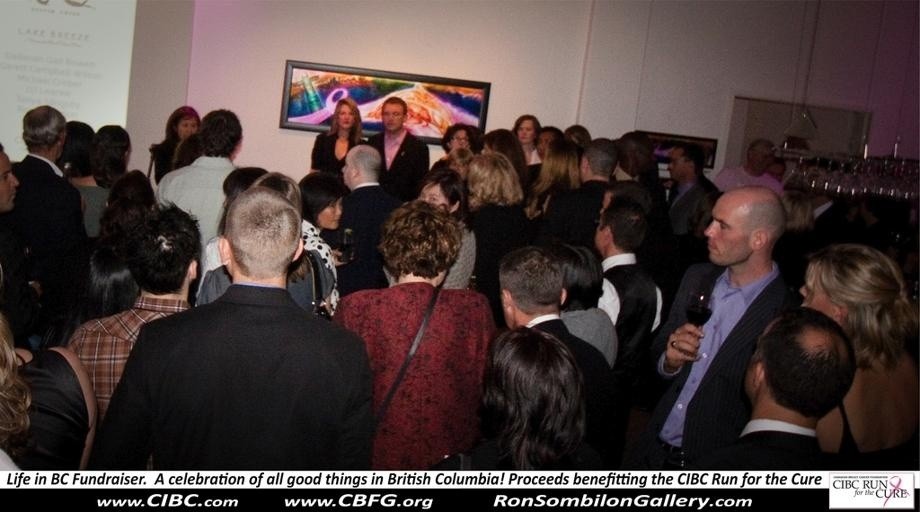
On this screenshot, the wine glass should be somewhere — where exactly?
[23,246,35,283]
[684,290,715,364]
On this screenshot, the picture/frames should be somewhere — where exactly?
[278,59,491,151]
[633,128,720,171]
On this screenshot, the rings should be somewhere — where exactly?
[671,341,676,348]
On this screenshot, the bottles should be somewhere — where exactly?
[785,154,920,201]
[337,228,355,263]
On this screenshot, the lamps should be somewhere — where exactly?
[780,0,823,143]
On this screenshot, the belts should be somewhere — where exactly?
[646,432,688,468]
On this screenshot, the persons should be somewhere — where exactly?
[148,107,201,195]
[0,98,920,469]
[310,99,366,180]
[156,109,243,299]
[364,99,430,203]
